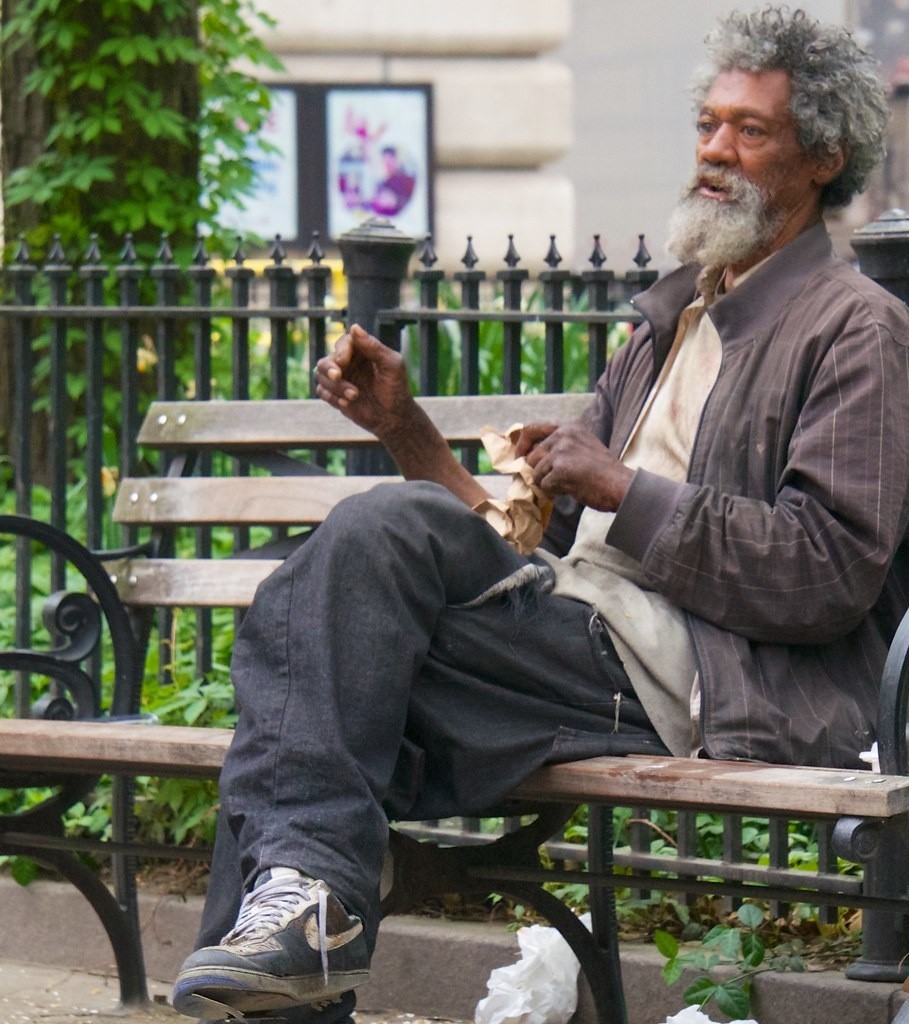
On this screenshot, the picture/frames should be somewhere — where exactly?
[313,81,434,249]
[193,83,312,251]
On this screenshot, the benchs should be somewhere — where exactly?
[0,391,908,1024]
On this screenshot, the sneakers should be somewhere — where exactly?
[173,864,370,1020]
[201,987,356,1024]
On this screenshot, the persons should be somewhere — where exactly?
[369,145,416,215]
[170,6,909,1024]
